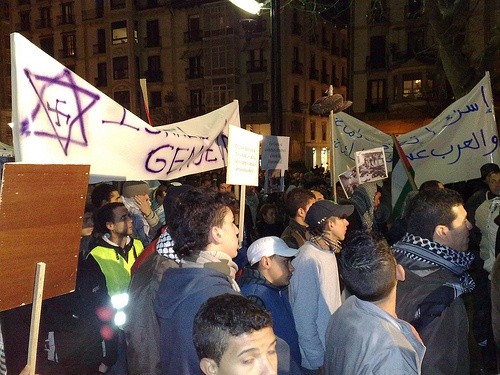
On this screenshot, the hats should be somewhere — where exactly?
[304,200,354,230]
[122,181,150,196]
[247,236,300,266]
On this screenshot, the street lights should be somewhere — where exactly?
[228,0,284,136]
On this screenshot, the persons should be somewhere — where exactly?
[193,294,278,375]
[391,187,475,375]
[0,158,500,375]
[324,234,427,375]
